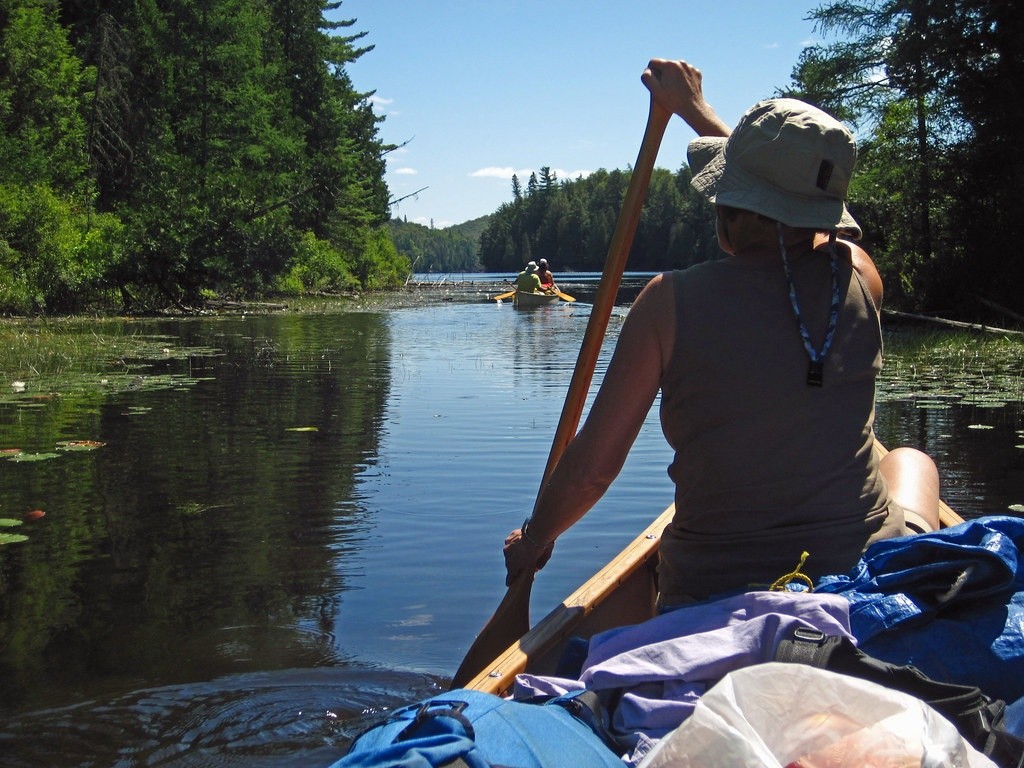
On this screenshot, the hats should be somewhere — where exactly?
[687,98,864,243]
[537,259,549,268]
[525,261,539,273]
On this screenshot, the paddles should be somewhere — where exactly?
[493,288,517,301]
[447,68,674,694]
[544,285,576,303]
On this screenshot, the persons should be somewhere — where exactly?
[534,259,554,288]
[508,261,547,295]
[504,59,941,616]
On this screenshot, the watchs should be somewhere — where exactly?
[522,516,550,549]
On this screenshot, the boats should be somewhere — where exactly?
[516,287,560,309]
